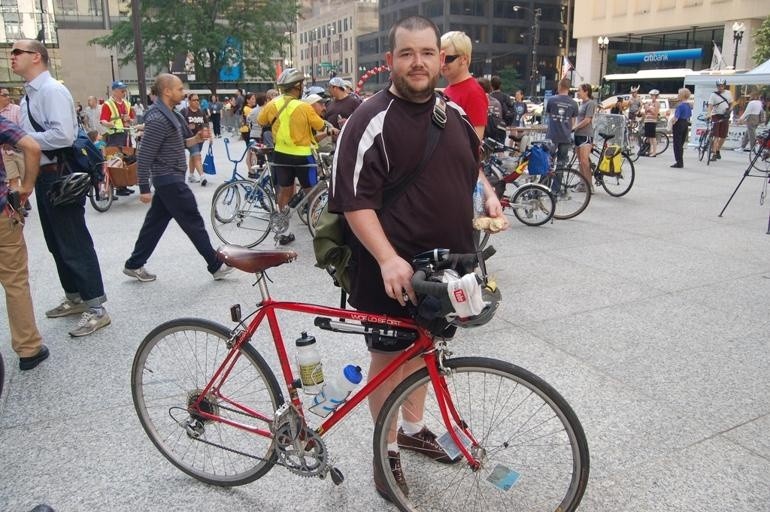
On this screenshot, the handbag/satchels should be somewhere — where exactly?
[596,145,621,177]
[203,154,216,175]
[57,127,105,185]
[528,146,549,174]
[313,201,361,294]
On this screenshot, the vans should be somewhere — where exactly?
[597,92,694,136]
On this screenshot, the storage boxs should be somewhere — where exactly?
[104,147,137,186]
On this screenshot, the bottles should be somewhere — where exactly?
[519,118,523,127]
[296,332,324,390]
[123,111,129,128]
[472,180,487,218]
[336,113,344,131]
[201,127,210,141]
[308,365,363,424]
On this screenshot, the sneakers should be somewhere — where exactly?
[645,153,657,156]
[279,232,295,245]
[709,151,721,161]
[123,265,156,282]
[201,176,208,186]
[46,299,90,317]
[397,426,464,464]
[571,184,594,195]
[68,307,111,336]
[248,171,257,178]
[733,147,743,152]
[373,451,409,503]
[188,176,200,182]
[670,163,684,168]
[212,261,237,281]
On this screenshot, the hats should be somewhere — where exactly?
[112,81,127,90]
[302,77,352,105]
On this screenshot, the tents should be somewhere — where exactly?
[683,58,770,151]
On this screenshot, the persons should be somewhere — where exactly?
[509,79,691,200]
[326,15,510,503]
[30,504,55,512]
[0,41,111,397]
[101,71,380,280]
[707,80,736,161]
[736,90,763,153]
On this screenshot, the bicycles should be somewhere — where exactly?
[86,123,145,214]
[696,114,723,167]
[749,116,770,173]
[124,243,592,512]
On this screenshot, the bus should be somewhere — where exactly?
[185,88,240,104]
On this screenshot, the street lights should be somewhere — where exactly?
[732,19,747,70]
[359,66,367,79]
[310,27,320,87]
[597,34,610,85]
[327,24,336,79]
[512,5,542,99]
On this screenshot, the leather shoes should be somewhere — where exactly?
[19,344,49,369]
[112,188,135,200]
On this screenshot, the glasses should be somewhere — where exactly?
[11,49,44,57]
[445,54,460,63]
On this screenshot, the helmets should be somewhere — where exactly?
[649,89,659,95]
[46,172,93,209]
[276,68,305,85]
[441,287,502,328]
[716,79,726,86]
